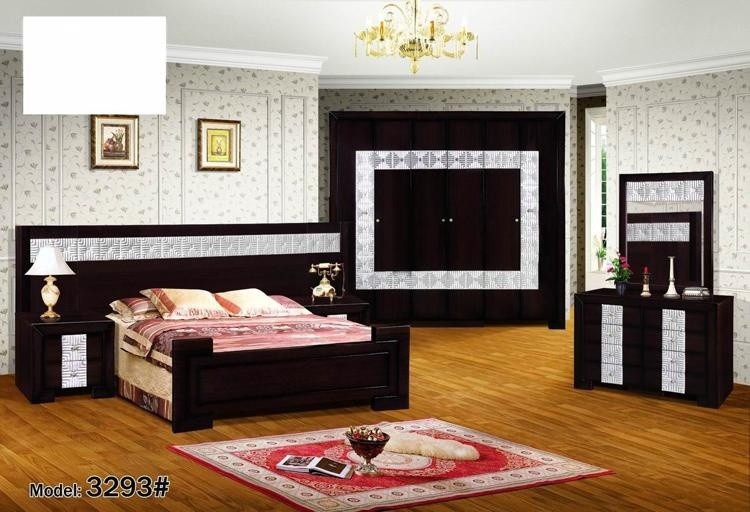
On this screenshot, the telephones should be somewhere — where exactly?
[309,262,344,303]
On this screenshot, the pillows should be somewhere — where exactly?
[109,288,311,322]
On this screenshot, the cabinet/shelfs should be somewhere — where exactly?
[645,292,734,409]
[574,288,645,393]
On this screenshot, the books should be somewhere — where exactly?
[275,454,357,479]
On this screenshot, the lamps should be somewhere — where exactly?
[26,245,76,322]
[353,0,479,75]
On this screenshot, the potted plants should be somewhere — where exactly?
[614,280,627,296]
[597,249,607,271]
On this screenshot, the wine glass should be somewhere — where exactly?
[345,432,390,473]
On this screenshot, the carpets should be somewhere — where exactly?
[164,416,617,512]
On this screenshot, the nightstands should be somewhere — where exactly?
[18,312,116,405]
[299,289,369,326]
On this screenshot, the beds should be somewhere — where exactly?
[100,307,410,433]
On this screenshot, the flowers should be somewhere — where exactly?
[605,251,633,283]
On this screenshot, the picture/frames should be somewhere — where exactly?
[198,118,241,172]
[90,114,140,170]
